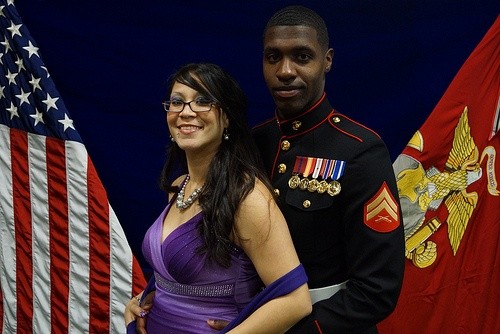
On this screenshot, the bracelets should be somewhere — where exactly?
[134,297,141,305]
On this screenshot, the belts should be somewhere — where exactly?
[309,281,349,307]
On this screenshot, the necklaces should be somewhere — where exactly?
[175,174,208,210]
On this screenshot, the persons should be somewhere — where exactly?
[123,61,314,334]
[135,6,408,334]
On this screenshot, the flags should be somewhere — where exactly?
[1,0,149,334]
[374,11,500,334]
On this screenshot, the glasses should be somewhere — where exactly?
[162,99,219,112]
